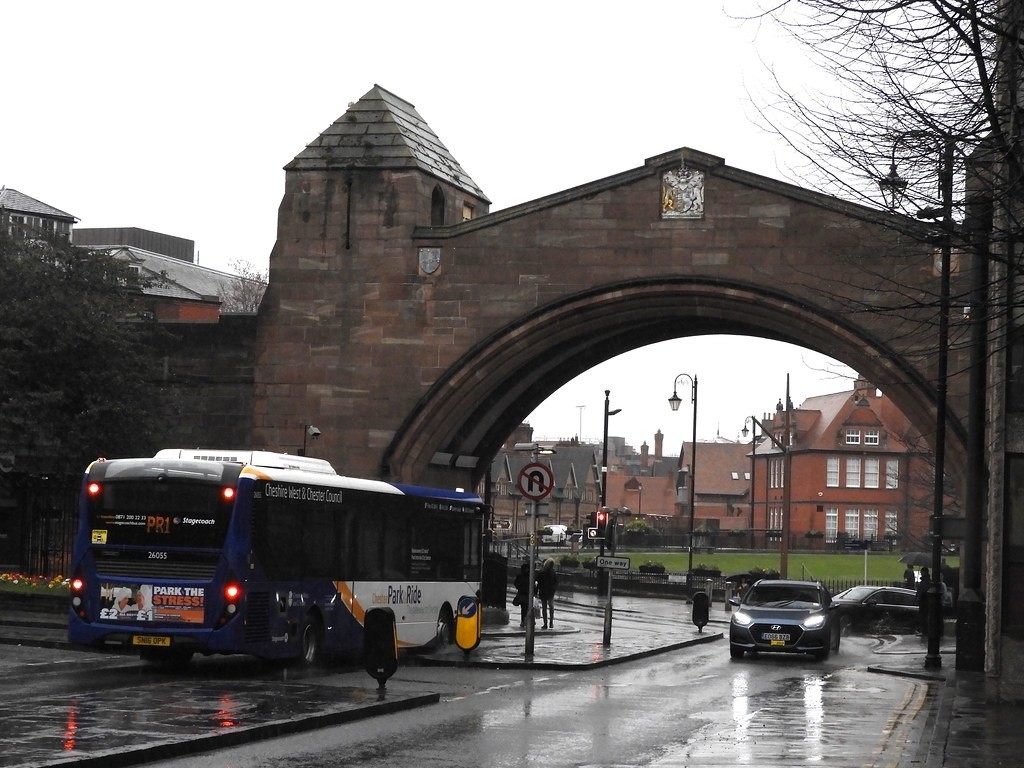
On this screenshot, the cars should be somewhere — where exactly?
[830,586,924,637]
[730,577,841,661]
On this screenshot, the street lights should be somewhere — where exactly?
[602,390,623,509]
[877,128,954,672]
[742,416,791,580]
[514,442,557,657]
[638,482,644,520]
[668,373,695,604]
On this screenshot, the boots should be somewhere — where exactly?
[541,611,547,629]
[549,612,554,628]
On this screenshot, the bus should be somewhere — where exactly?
[66,450,495,669]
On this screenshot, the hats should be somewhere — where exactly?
[921,567,929,573]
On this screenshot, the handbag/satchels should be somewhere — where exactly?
[533,597,540,619]
[512,592,519,606]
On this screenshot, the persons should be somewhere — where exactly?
[913,558,952,637]
[903,564,916,590]
[514,558,560,629]
[735,581,752,600]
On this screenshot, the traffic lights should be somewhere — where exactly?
[597,512,626,549]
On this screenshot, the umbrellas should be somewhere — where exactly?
[723,572,753,582]
[898,552,934,583]
